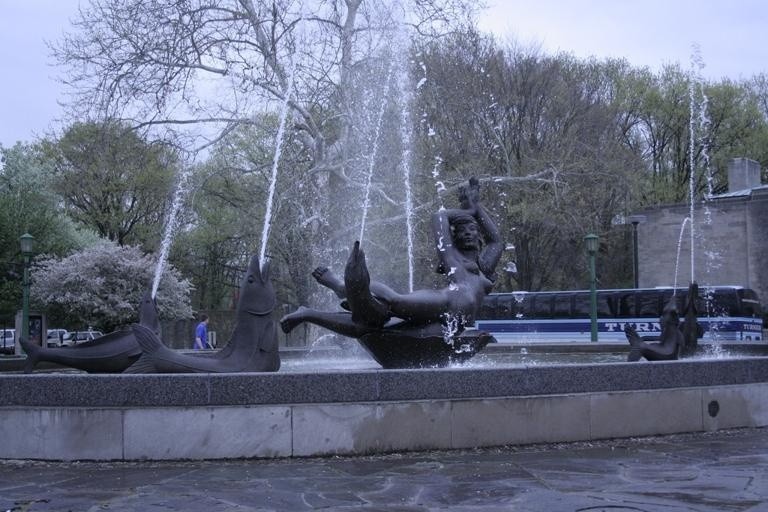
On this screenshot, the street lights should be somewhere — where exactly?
[584,233,600,342]
[18,233,35,355]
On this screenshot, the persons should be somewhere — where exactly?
[279,170,505,336]
[191,314,213,350]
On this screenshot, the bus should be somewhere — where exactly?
[474,285,763,343]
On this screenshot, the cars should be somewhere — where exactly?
[47,328,104,348]
[0,329,16,355]
